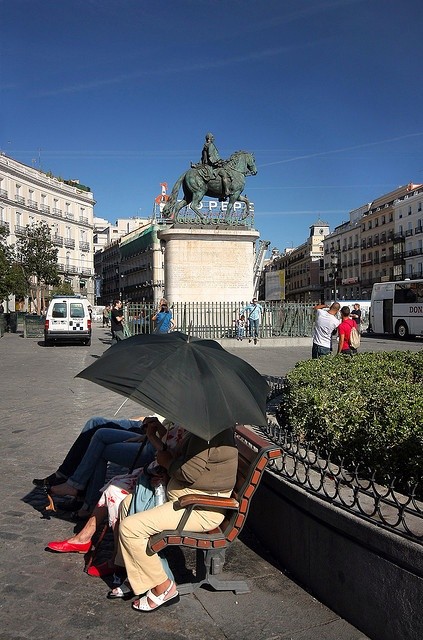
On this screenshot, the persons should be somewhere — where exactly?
[336,306,360,358]
[232,315,245,340]
[151,298,175,334]
[349,304,361,334]
[111,299,124,345]
[311,302,340,359]
[202,132,229,203]
[239,298,263,341]
[102,305,112,328]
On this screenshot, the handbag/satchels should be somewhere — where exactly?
[151,326,159,335]
[128,470,168,515]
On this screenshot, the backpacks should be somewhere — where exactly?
[344,320,360,349]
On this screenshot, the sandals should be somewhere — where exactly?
[108,576,134,599]
[132,581,180,613]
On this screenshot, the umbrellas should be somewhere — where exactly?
[76,323,272,475]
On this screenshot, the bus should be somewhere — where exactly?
[369,279,423,339]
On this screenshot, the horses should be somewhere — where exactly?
[162,150,257,225]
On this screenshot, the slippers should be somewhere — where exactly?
[71,511,91,519]
[42,480,58,512]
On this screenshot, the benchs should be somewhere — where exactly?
[124,414,284,597]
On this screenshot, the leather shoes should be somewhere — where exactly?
[48,538,92,554]
[88,558,116,577]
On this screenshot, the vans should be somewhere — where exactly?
[45,296,91,346]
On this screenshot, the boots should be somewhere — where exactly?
[222,178,234,197]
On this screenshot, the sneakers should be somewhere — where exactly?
[240,337,243,340]
[33,473,66,488]
[249,337,253,341]
[237,336,239,340]
[255,337,259,341]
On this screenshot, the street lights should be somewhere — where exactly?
[94,274,100,306]
[119,271,126,304]
[330,249,338,304]
